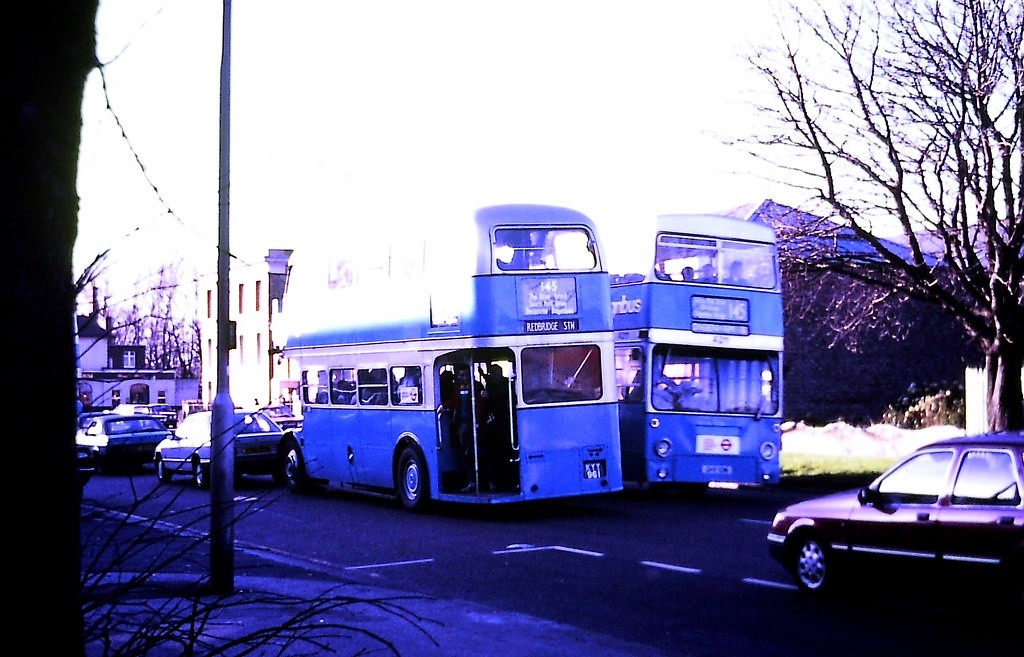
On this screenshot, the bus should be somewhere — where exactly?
[542,209,783,493]
[274,201,623,511]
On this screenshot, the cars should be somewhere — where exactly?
[150,409,288,488]
[76,402,179,470]
[258,407,305,433]
[762,432,1024,608]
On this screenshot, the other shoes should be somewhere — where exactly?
[459,483,475,494]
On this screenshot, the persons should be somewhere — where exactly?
[655,261,672,280]
[632,353,678,391]
[255,398,259,405]
[439,363,514,492]
[681,267,694,281]
[723,261,748,284]
[316,368,422,405]
[700,264,718,281]
